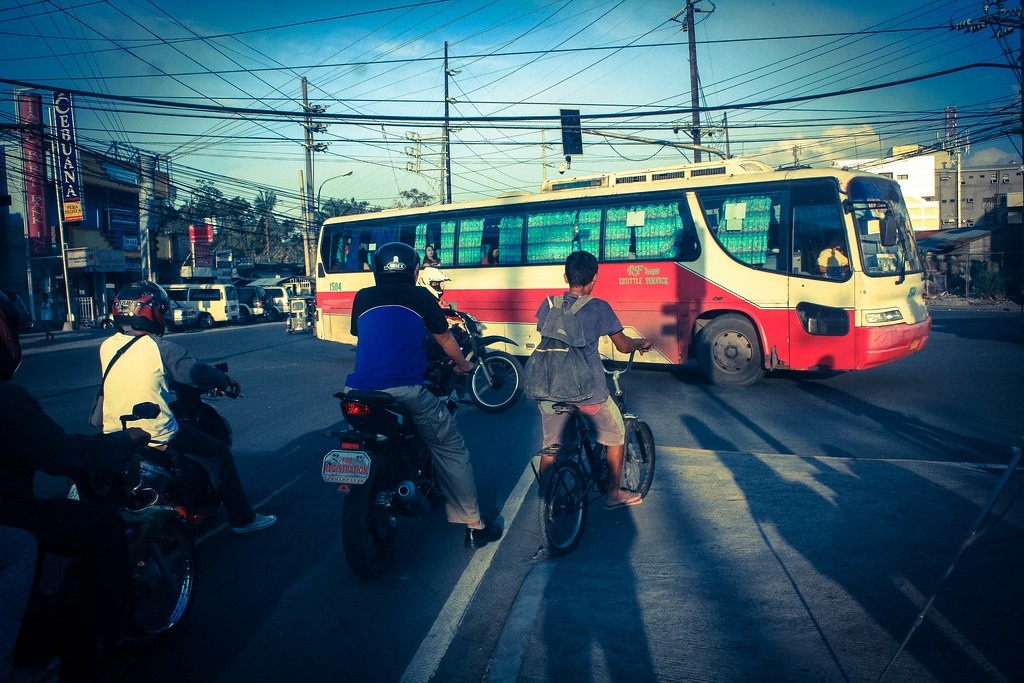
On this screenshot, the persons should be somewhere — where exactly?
[816,230,848,273]
[422,245,441,267]
[343,242,502,551]
[481,244,499,265]
[40,293,56,344]
[420,266,483,328]
[99,280,278,534]
[345,237,352,256]
[520,252,654,510]
[0,289,150,683]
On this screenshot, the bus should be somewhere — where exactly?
[314,157,932,389]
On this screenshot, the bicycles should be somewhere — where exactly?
[531,347,656,556]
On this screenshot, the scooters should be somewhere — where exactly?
[5,362,245,683]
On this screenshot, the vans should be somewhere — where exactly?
[94,283,316,336]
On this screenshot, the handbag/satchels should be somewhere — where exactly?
[87,390,103,427]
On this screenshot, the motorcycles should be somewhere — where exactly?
[322,355,475,581]
[431,304,524,413]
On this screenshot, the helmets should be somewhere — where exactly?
[417,266,450,301]
[372,243,421,286]
[0,292,22,384]
[114,281,171,339]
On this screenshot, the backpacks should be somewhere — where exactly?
[524,296,596,402]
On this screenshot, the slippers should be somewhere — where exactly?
[602,490,643,510]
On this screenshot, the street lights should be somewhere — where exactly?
[317,170,352,248]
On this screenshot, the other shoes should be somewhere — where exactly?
[97,633,154,656]
[465,517,502,550]
[230,513,277,534]
